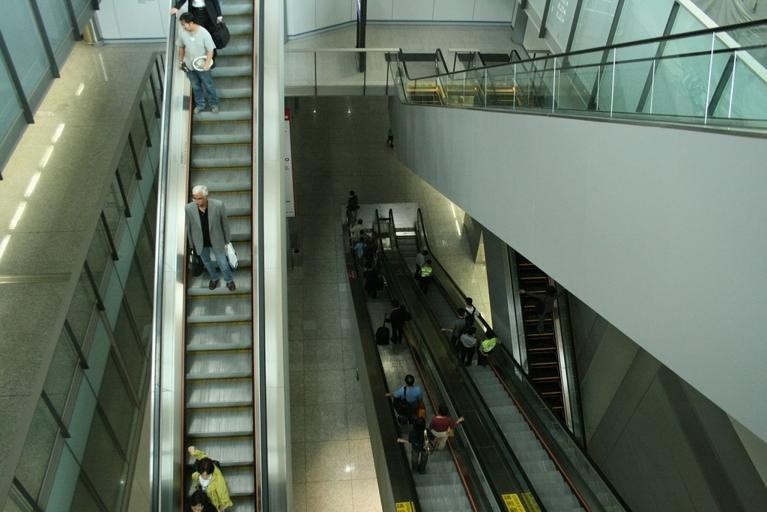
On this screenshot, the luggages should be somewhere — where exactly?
[376,326,390,346]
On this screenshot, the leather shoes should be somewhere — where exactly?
[226,282,236,292]
[208,280,220,290]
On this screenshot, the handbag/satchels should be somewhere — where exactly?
[224,243,239,271]
[393,398,412,419]
[417,402,427,418]
[448,427,454,438]
[423,442,434,455]
[187,255,203,277]
[212,21,230,50]
[402,311,410,323]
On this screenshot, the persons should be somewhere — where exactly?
[385,126,396,149]
[439,296,504,369]
[184,184,237,292]
[383,297,409,346]
[396,417,437,475]
[175,10,221,115]
[384,374,425,426]
[428,401,465,453]
[186,447,235,512]
[521,283,570,337]
[186,487,218,512]
[340,181,434,294]
[169,0,230,71]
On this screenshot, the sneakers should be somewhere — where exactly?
[194,107,204,114]
[211,105,220,113]
